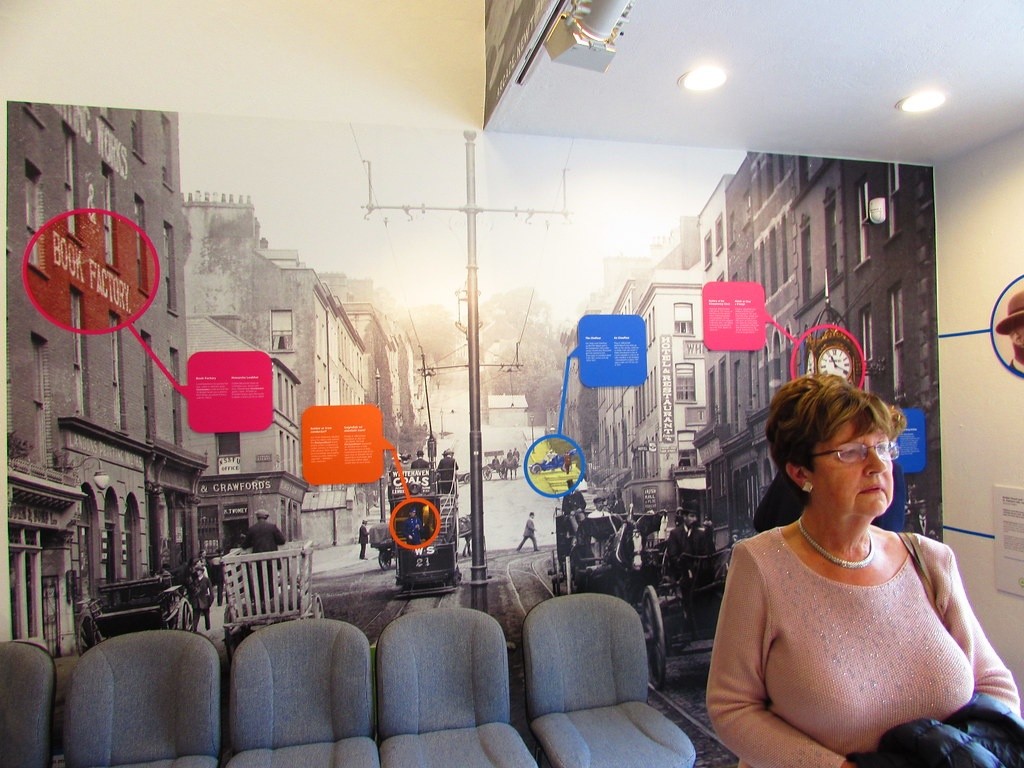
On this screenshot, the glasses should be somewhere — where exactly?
[811,440,902,464]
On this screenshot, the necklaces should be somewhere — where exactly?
[797,516,876,568]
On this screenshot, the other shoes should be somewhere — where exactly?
[534,549,539,551]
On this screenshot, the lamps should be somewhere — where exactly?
[48,456,109,489]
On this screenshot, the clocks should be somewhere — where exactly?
[806,329,861,394]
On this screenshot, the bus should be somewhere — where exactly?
[387,462,460,600]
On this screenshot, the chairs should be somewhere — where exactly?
[222,618,379,768]
[375,607,538,768]
[64,631,222,768]
[0,641,58,768]
[521,592,696,768]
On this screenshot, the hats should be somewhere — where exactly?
[416,451,424,456]
[593,497,606,504]
[192,558,200,565]
[194,566,204,572]
[566,479,574,488]
[442,450,451,455]
[677,499,700,514]
[255,509,269,519]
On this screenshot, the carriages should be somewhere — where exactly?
[77,556,207,660]
[484,456,520,482]
[367,511,472,569]
[549,510,661,600]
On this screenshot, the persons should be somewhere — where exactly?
[516,512,540,552]
[704,375,1024,768]
[358,519,369,559]
[187,447,727,632]
[243,507,286,601]
